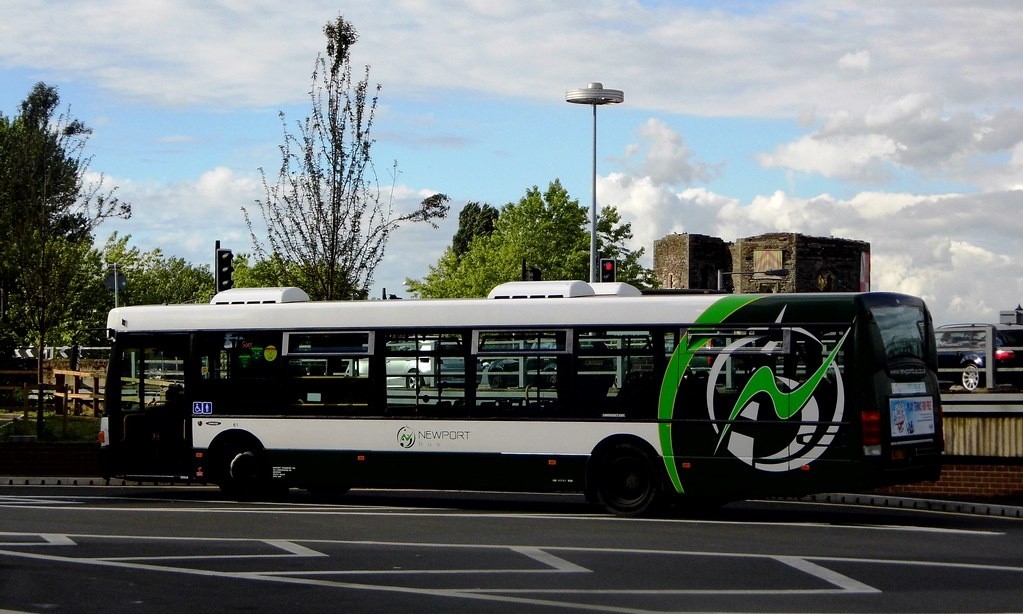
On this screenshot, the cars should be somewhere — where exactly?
[343,334,483,388]
[0,415,39,441]
[488,337,616,390]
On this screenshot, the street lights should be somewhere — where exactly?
[565,83,624,283]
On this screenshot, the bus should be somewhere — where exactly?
[98,276,948,519]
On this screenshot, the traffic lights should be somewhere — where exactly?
[217,253,234,291]
[600,258,617,282]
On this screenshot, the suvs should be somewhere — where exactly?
[934,322,1023,391]
[624,334,724,375]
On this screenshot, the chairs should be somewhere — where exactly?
[951,337,963,343]
[435,399,550,414]
